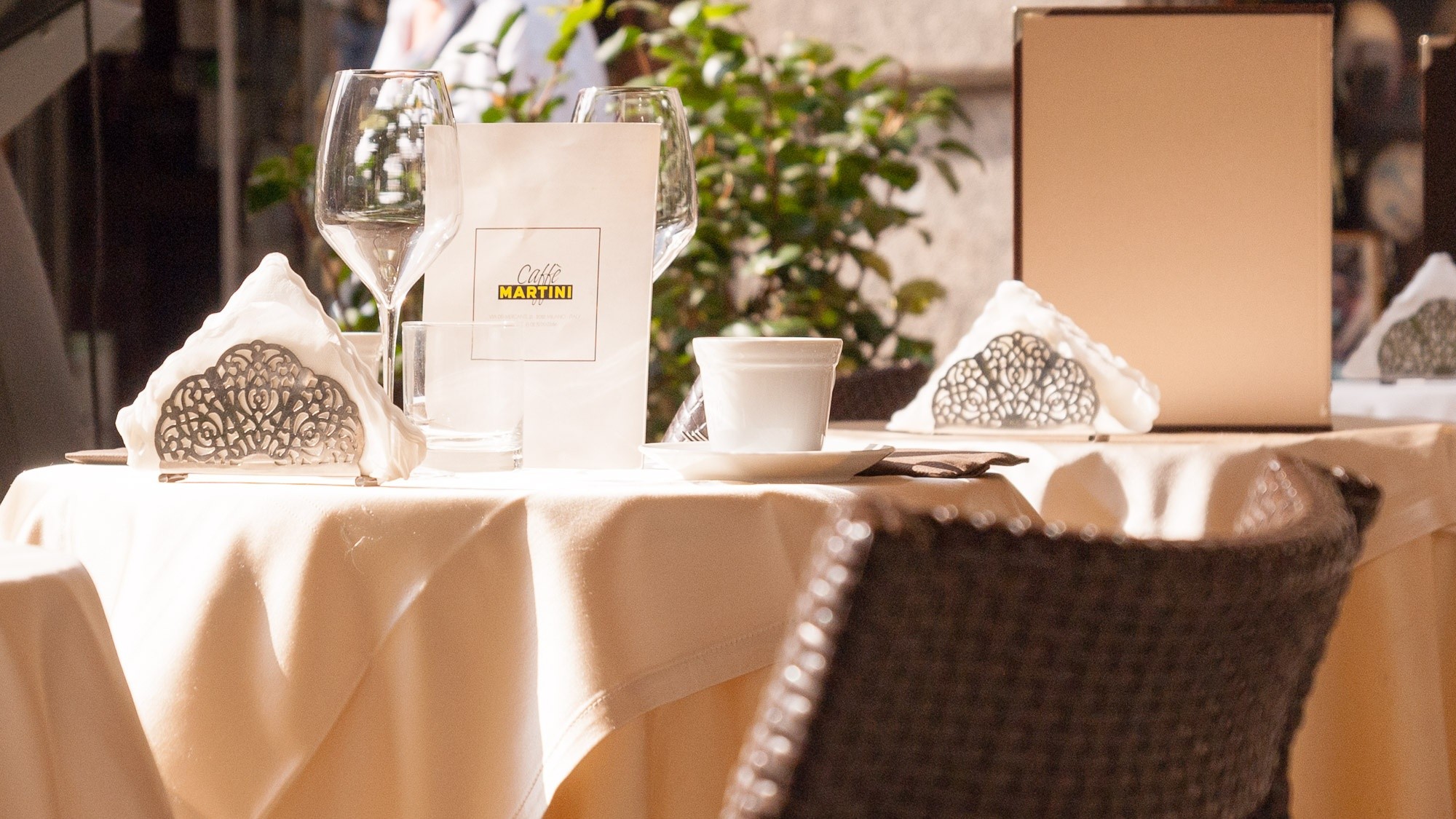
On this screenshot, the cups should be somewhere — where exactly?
[570,86,698,283]
[334,333,382,379]
[691,337,844,453]
[399,321,523,471]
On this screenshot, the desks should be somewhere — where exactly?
[1331,377,1456,421]
[0,416,1456,818]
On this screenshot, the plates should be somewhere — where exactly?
[63,448,128,463]
[642,441,894,481]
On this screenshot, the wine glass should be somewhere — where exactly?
[315,69,457,401]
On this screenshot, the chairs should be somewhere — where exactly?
[718,445,1386,819]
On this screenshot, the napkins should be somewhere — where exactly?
[884,281,1162,437]
[1344,252,1456,382]
[115,253,423,480]
